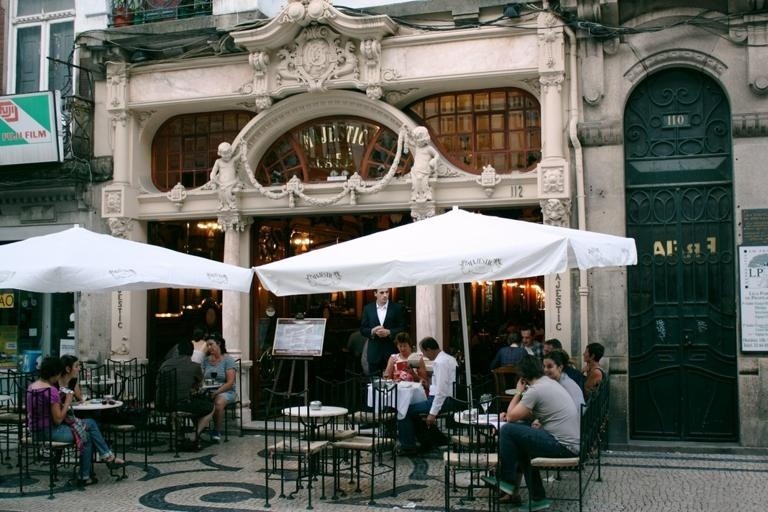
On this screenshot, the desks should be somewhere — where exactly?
[367,377,428,419]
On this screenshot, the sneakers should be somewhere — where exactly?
[481,475,514,495]
[518,499,551,512]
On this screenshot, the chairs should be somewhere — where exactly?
[311,376,362,487]
[263,387,328,510]
[4,358,244,499]
[331,382,398,507]
[419,359,608,511]
[345,369,395,467]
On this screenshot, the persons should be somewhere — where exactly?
[195,336,238,441]
[191,328,207,364]
[53,354,86,403]
[383,332,429,400]
[393,337,459,455]
[25,356,133,484]
[154,340,216,447]
[360,288,408,384]
[476,323,607,512]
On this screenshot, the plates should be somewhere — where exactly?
[399,383,412,388]
[90,399,102,403]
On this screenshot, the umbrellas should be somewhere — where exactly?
[0,224,254,385]
[252,205,638,410]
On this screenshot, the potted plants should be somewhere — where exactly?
[107,1,148,28]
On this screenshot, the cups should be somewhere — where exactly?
[205,379,212,385]
[464,411,474,421]
[310,401,322,410]
[393,372,400,381]
[104,394,112,402]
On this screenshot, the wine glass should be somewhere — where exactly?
[383,371,389,384]
[480,396,492,419]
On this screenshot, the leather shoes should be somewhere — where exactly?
[84,477,98,486]
[105,460,133,470]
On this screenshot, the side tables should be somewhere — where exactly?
[281,406,349,476]
